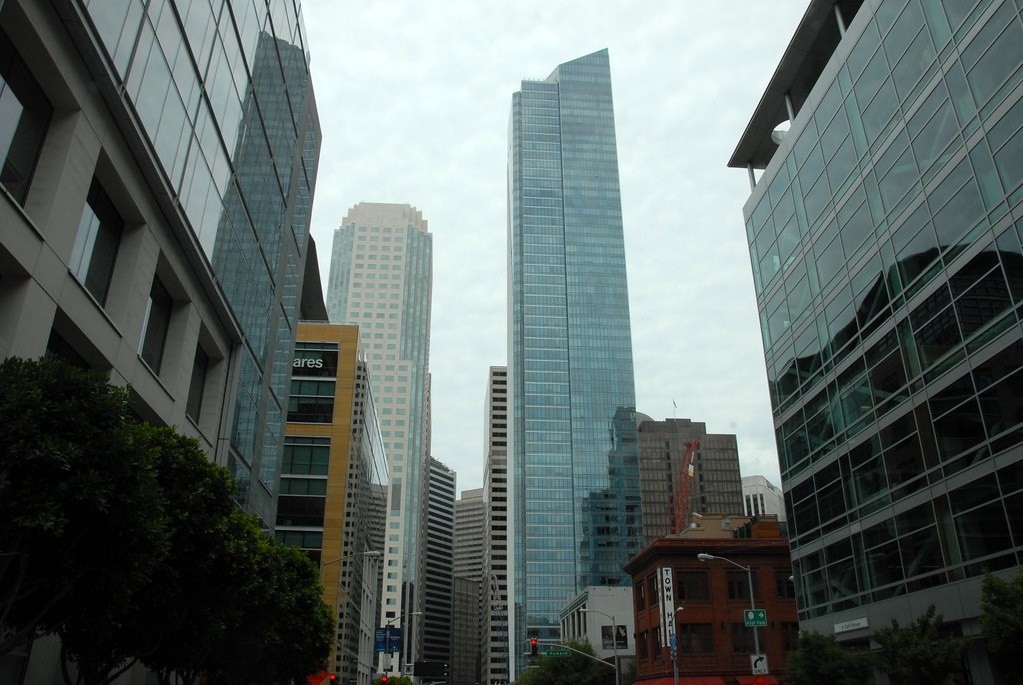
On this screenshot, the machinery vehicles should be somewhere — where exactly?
[667,436,701,533]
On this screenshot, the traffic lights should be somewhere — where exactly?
[330,674,337,683]
[530,638,538,656]
[382,674,387,683]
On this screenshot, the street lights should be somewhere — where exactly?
[696,552,761,655]
[579,608,619,685]
[672,607,684,684]
[385,612,422,681]
